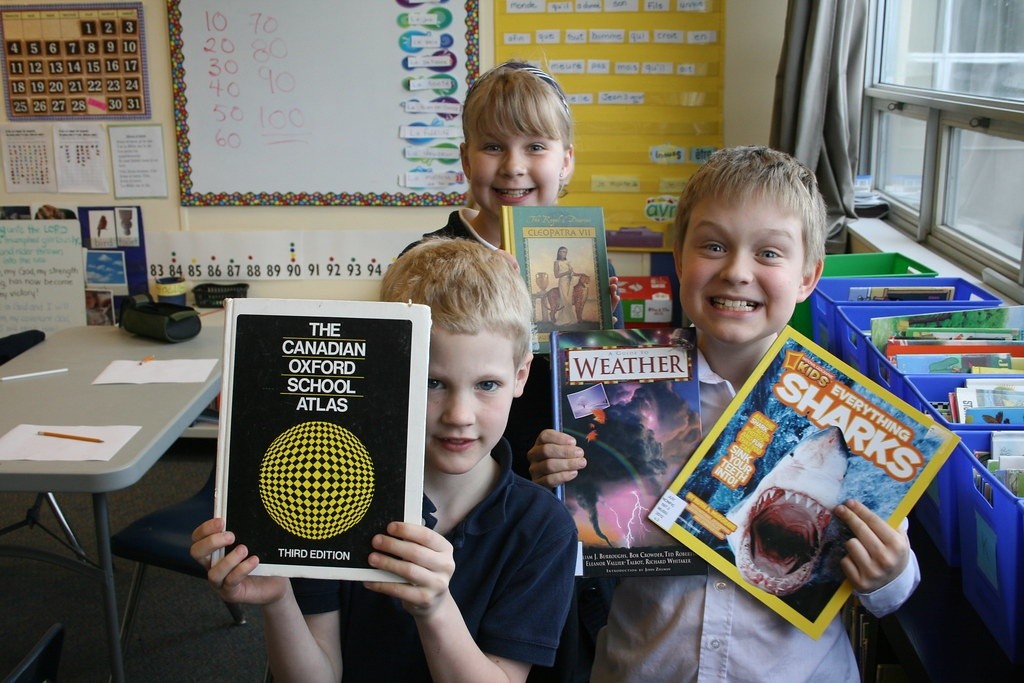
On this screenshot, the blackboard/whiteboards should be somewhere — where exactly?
[167,0,480,208]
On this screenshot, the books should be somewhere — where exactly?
[209,297,434,583]
[847,286,1024,425]
[973,431,1024,506]
[500,205,613,354]
[189,63,921,683]
[648,324,961,640]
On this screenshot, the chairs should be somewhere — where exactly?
[0,329,88,563]
[109,462,247,682]
[1,621,63,683]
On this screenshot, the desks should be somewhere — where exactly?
[895,513,1023,683]
[0,312,223,683]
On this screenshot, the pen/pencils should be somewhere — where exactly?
[139,355,156,365]
[2,366,69,382]
[38,431,104,442]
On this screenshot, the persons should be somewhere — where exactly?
[551,327,708,577]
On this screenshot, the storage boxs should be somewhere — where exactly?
[787,252,1024,666]
[620,276,672,323]
